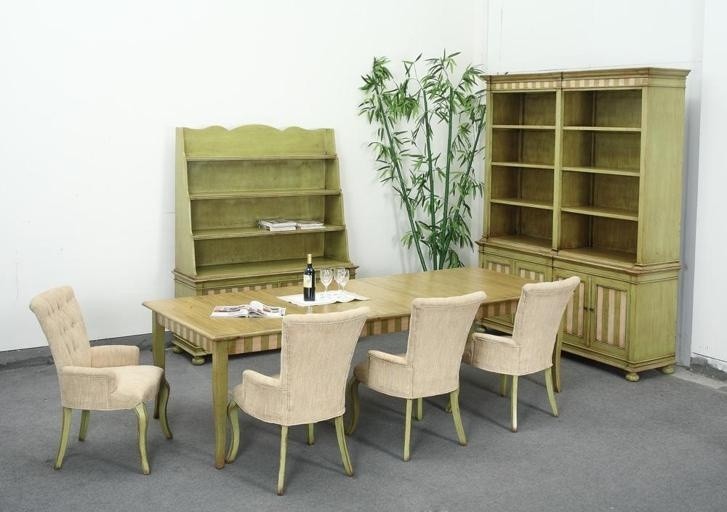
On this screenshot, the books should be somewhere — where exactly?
[257,215,325,232]
[210,300,287,320]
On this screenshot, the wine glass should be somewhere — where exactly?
[320,265,351,297]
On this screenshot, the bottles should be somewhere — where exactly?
[302,253,317,301]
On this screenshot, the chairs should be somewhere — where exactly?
[444,275,581,433]
[29,285,173,475]
[225,306,370,496]
[345,290,487,463]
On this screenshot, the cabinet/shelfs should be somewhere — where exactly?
[474,66,692,383]
[171,123,359,365]
